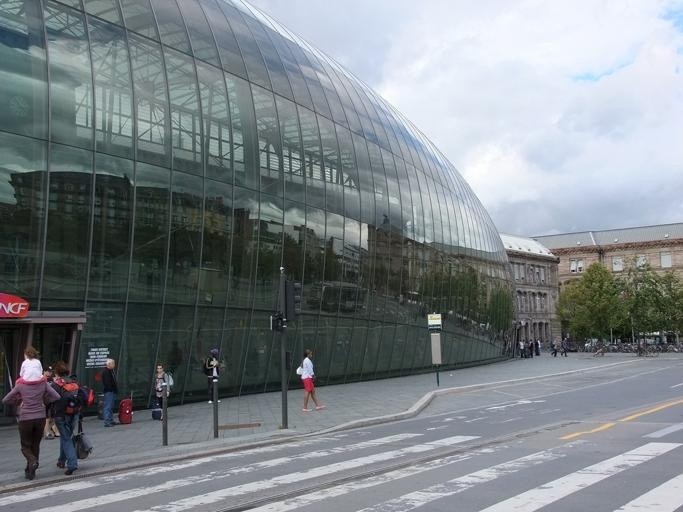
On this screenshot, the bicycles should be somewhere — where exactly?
[590,342,682,356]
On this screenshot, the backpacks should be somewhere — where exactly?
[163,372,174,390]
[53,378,81,414]
[79,385,94,407]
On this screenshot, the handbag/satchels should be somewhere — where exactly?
[72,432,92,459]
[297,367,303,376]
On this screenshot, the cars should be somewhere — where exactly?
[293,282,301,302]
[139,253,176,286]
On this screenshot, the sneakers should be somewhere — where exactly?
[208,400,212,404]
[302,408,312,412]
[316,406,326,409]
[65,468,77,475]
[218,400,221,403]
[105,421,118,427]
[26,463,38,479]
[57,463,64,468]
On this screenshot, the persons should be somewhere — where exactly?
[299,348,325,413]
[518,337,567,359]
[486,332,510,358]
[13,345,50,406]
[44,360,93,475]
[1,381,62,481]
[167,338,182,373]
[592,340,604,356]
[203,347,222,404]
[101,357,119,427]
[154,363,170,420]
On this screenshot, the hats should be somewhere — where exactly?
[211,349,218,358]
[43,366,55,372]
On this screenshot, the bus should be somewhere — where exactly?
[308,280,369,312]
[637,331,679,346]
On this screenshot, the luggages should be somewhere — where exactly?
[152,400,162,419]
[119,391,133,424]
[97,395,104,419]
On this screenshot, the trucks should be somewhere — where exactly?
[71,250,112,283]
